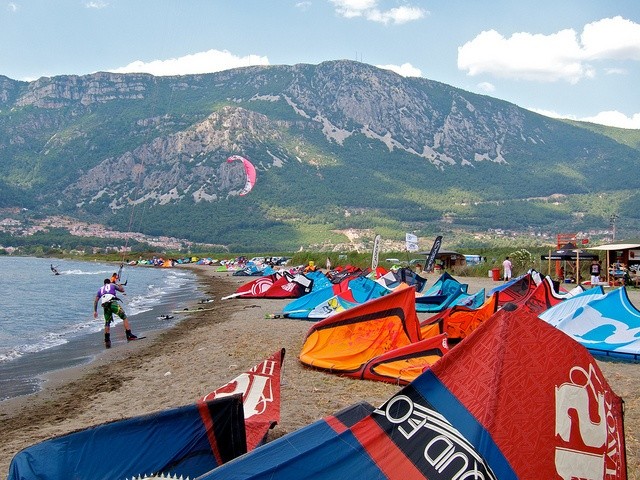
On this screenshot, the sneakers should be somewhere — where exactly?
[105,332,110,342]
[126,329,137,338]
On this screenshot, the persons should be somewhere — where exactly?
[93,278,137,342]
[110,273,120,283]
[50,264,59,276]
[589,258,600,288]
[558,263,583,282]
[326,257,331,271]
[599,260,606,282]
[629,270,640,288]
[502,257,513,284]
